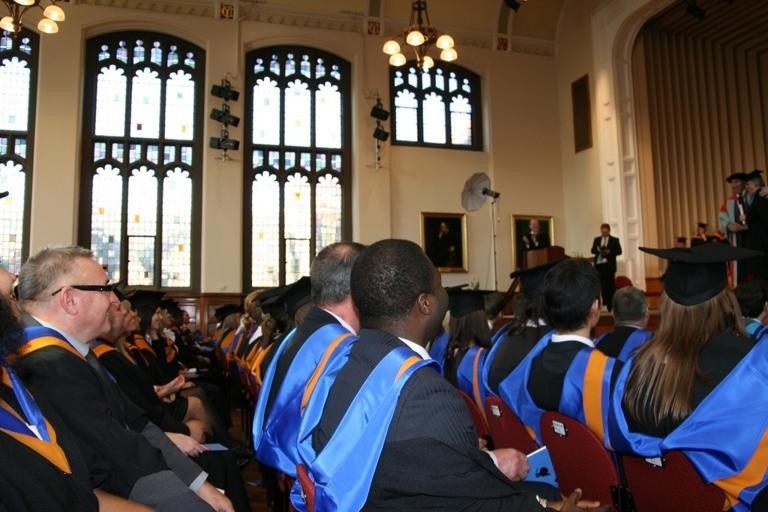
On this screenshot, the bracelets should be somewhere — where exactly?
[540,498,546,508]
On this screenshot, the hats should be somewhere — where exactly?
[638,243,763,305]
[490,261,555,315]
[250,274,312,320]
[727,168,762,180]
[442,284,494,318]
[128,289,179,313]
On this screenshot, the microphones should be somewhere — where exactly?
[738,204,746,224]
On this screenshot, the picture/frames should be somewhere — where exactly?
[417,209,469,275]
[508,213,556,280]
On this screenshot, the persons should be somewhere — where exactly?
[252,241,530,481]
[594,286,656,364]
[498,258,624,453]
[520,219,550,251]
[677,237,686,248]
[717,169,768,319]
[88,279,224,488]
[612,241,767,512]
[425,260,562,411]
[696,222,708,241]
[591,224,622,294]
[1,265,154,512]
[289,239,601,512]
[12,246,232,512]
[214,276,311,387]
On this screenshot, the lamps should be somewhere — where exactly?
[379,1,458,75]
[0,0,66,38]
[370,97,391,170]
[203,77,242,159]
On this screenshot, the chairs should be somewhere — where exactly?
[484,394,540,458]
[295,464,315,512]
[220,349,261,444]
[455,384,485,440]
[535,409,620,510]
[618,434,729,510]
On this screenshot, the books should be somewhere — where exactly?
[521,446,559,489]
